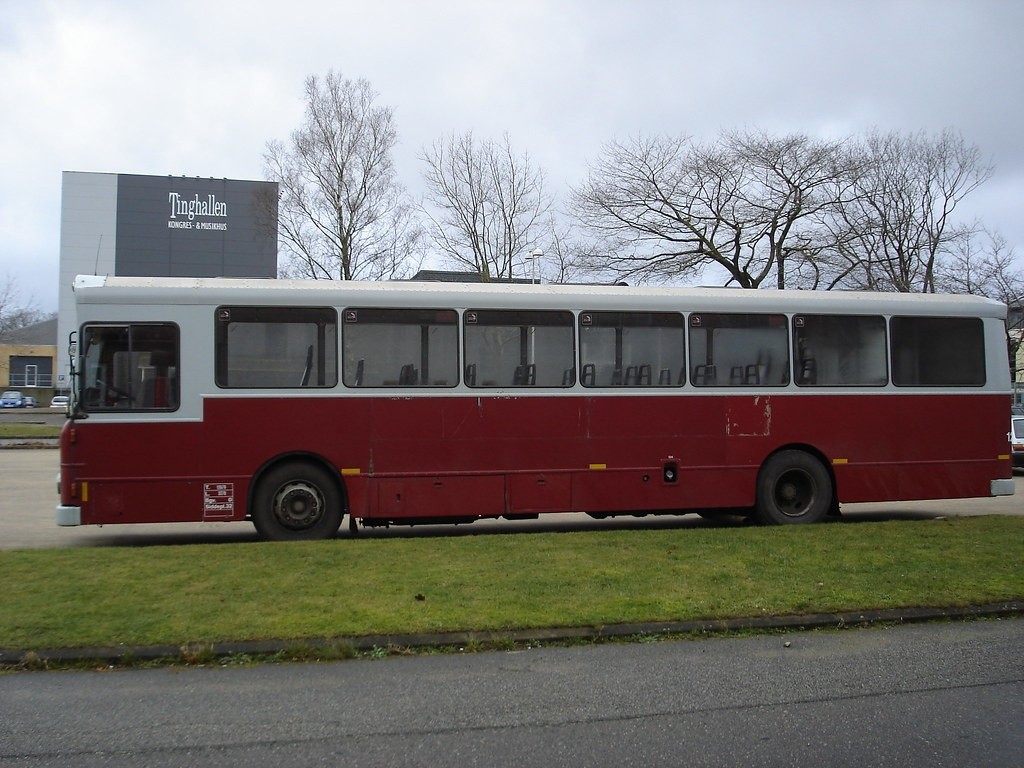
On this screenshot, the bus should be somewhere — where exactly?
[56,272,1017,542]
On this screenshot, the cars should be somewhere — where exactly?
[49,395,70,408]
[1011,414,1024,468]
[0,390,27,408]
[25,396,41,408]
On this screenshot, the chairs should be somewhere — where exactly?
[355,356,421,387]
[467,362,477,386]
[513,362,537,386]
[658,369,685,385]
[730,364,760,386]
[694,364,717,386]
[300,344,315,386]
[562,362,596,387]
[612,365,651,385]
[798,357,816,384]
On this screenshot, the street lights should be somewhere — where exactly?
[525,248,546,284]
